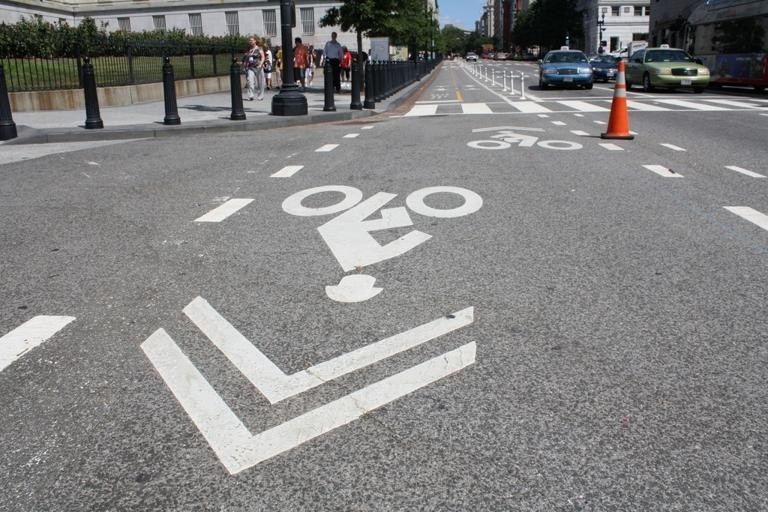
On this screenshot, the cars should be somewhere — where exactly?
[464,49,509,62]
[623,43,711,95]
[583,39,649,83]
[537,46,595,91]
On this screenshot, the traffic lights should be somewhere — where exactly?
[565,31,570,46]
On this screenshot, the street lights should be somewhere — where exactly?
[596,11,606,55]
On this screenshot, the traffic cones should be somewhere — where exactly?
[600,60,636,142]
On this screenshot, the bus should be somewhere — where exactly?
[683,0,768,100]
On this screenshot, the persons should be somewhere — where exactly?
[243,29,353,102]
